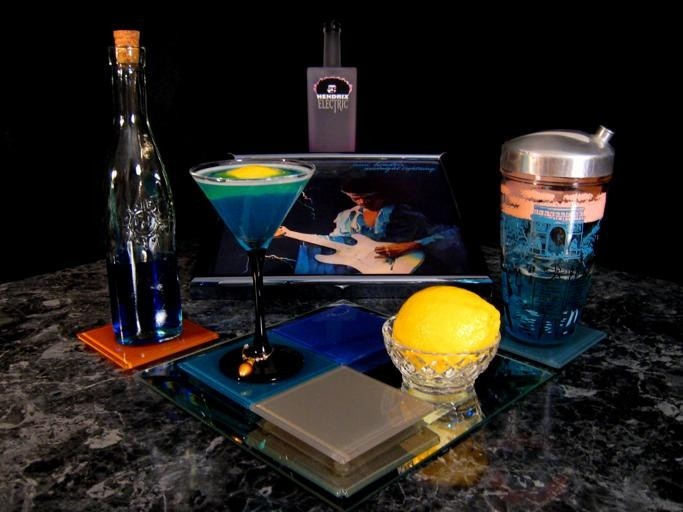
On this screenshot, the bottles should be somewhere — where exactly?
[100,29,184,347]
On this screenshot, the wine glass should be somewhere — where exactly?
[395,391,493,488]
[379,312,506,405]
[187,157,318,385]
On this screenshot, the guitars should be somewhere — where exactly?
[272,225,426,274]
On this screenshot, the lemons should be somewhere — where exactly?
[391,285,502,374]
[227,165,279,180]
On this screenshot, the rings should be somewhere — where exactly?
[385,248,389,251]
[386,252,390,257]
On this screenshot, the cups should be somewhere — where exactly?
[496,125,619,348]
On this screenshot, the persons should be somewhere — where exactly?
[273,172,467,275]
